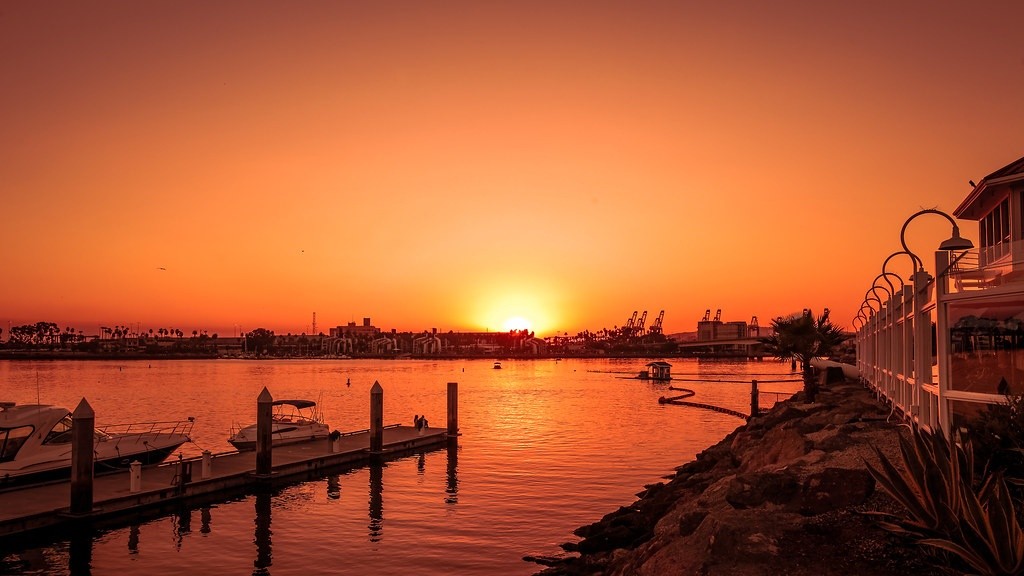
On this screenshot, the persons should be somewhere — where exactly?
[419,414,428,428]
[414,415,419,427]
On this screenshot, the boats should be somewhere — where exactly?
[493,362,502,369]
[227,397,332,451]
[0,401,194,488]
